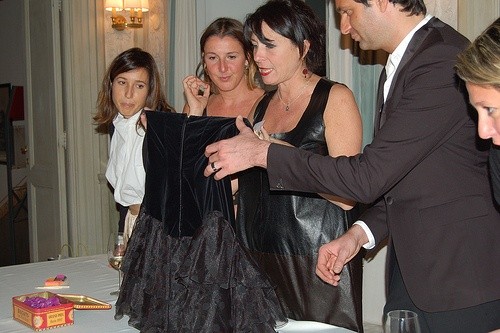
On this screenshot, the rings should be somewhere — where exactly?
[211,161,218,172]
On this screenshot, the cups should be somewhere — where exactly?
[384,310,421,333]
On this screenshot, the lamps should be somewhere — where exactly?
[104,0,149,30]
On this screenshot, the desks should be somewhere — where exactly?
[0,255,357,333]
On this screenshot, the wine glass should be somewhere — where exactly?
[107,232,129,296]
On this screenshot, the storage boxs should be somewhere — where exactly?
[13,292,74,330]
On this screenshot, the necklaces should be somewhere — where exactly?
[278,73,316,111]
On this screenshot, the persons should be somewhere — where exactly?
[454,18,500,209]
[203,0,500,333]
[245,0,363,333]
[90,47,176,267]
[184,16,266,220]
